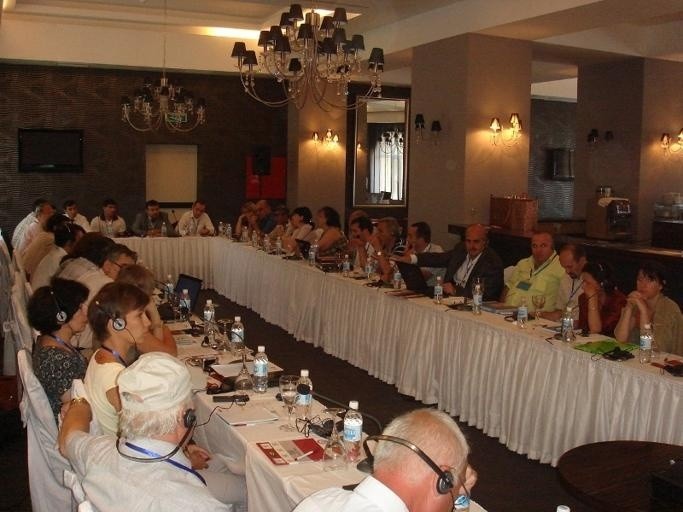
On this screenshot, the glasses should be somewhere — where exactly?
[439,463,471,507]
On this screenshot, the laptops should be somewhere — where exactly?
[395,261,444,297]
[157,274,203,320]
[295,239,311,259]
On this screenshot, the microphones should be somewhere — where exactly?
[66,323,79,351]
[172,210,182,237]
[297,384,384,474]
[125,328,137,351]
[181,307,253,364]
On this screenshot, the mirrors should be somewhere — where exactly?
[352,95,410,209]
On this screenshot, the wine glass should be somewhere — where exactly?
[218,319,234,355]
[323,406,346,467]
[235,349,254,390]
[532,294,546,321]
[335,252,342,275]
[204,304,219,342]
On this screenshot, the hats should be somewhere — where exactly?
[115,351,204,413]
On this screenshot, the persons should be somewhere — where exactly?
[389,224,682,356]
[9,197,478,511]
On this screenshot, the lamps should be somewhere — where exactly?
[121,0,208,142]
[311,129,340,149]
[375,123,406,154]
[586,128,614,154]
[231,0,384,113]
[489,113,522,151]
[660,128,683,159]
[414,114,443,147]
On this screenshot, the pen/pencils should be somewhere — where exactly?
[295,450,313,461]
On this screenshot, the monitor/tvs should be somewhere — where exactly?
[18,128,83,173]
[548,149,576,180]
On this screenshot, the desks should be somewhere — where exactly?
[110,235,218,288]
[217,233,683,465]
[143,289,488,512]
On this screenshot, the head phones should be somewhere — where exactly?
[200,334,222,348]
[117,408,197,462]
[48,287,68,323]
[364,435,453,494]
[61,223,75,240]
[504,312,534,322]
[94,297,127,331]
[50,216,57,231]
[590,261,613,294]
[603,346,632,361]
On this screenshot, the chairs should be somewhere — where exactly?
[58,472,95,511]
[16,348,77,511]
[0,232,35,377]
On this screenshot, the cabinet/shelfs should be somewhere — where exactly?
[651,218,682,310]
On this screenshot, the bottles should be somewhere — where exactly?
[308,245,315,267]
[639,324,654,365]
[434,276,444,304]
[343,255,350,277]
[472,277,484,315]
[231,317,244,352]
[161,222,167,237]
[394,266,402,289]
[254,346,269,392]
[165,275,190,322]
[203,300,215,341]
[218,221,282,256]
[343,401,363,463]
[560,306,574,336]
[296,369,313,421]
[517,298,528,329]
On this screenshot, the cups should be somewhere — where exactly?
[280,374,299,431]
